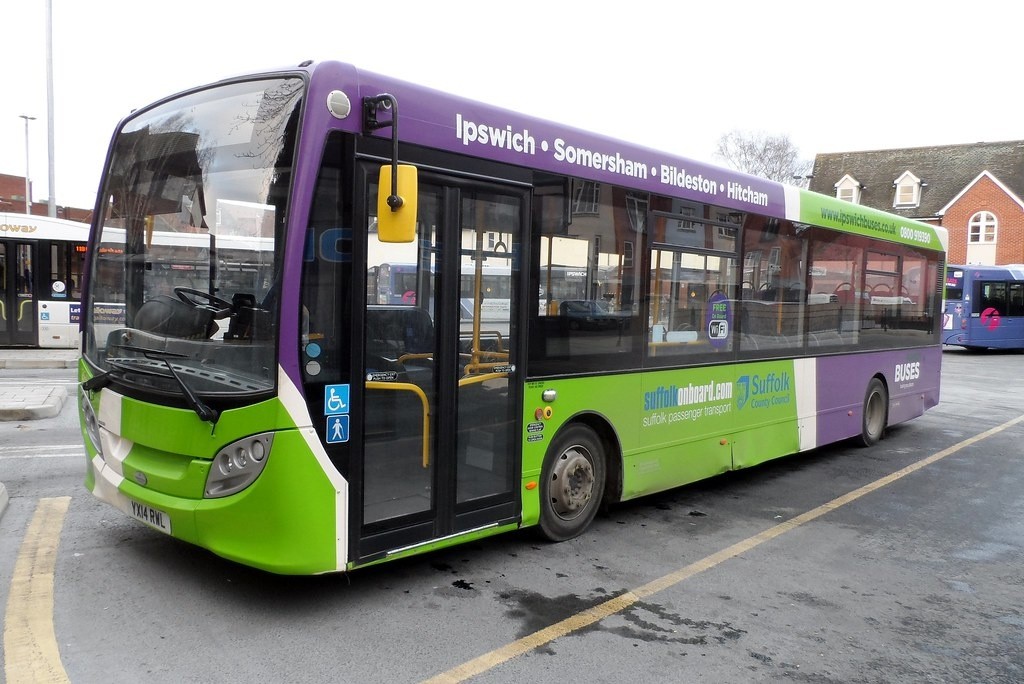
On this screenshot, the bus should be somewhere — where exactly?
[374,264,547,327]
[77,58,951,569]
[0,211,277,346]
[901,264,1024,354]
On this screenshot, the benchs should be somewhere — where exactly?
[366,305,433,354]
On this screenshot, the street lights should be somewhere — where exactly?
[792,174,816,186]
[20,115,38,216]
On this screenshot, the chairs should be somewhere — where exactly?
[738,280,755,300]
[868,284,892,303]
[892,285,909,297]
[832,282,856,304]
[708,289,728,300]
[864,283,872,304]
[760,280,807,302]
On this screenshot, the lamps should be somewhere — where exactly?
[859,183,867,190]
[891,179,896,188]
[917,180,928,186]
